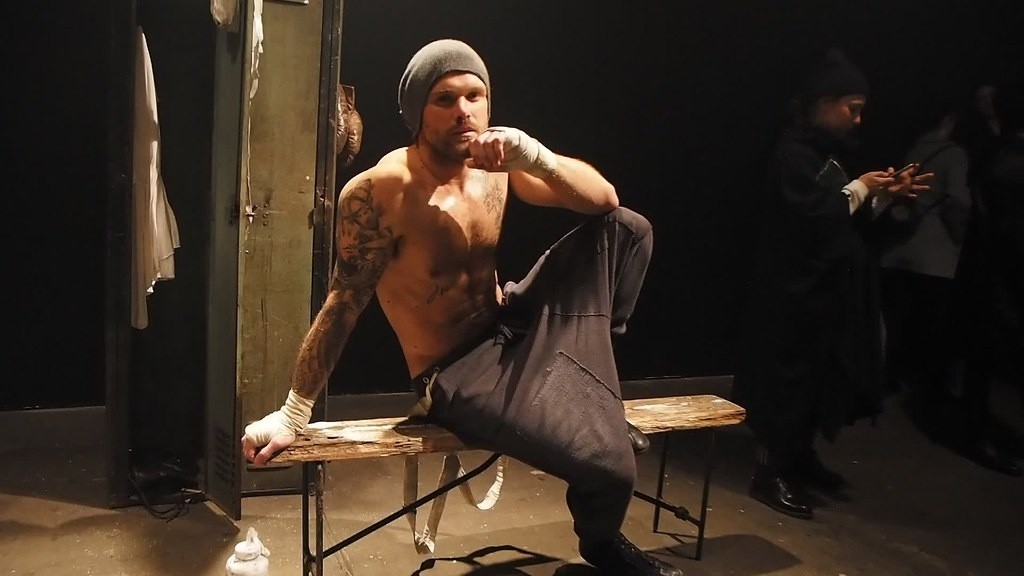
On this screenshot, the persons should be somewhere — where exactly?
[652,70,935,521]
[238,38,682,576]
[878,66,1024,480]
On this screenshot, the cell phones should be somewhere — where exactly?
[888,163,915,178]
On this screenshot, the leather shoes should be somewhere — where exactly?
[751,472,813,517]
[811,468,845,492]
[627,421,650,453]
[578,530,683,576]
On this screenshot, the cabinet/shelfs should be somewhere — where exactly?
[104,0,348,509]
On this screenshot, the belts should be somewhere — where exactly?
[411,326,496,397]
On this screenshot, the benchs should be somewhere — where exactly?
[247,393,747,575]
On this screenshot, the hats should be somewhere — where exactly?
[399,40,492,139]
[803,46,870,98]
